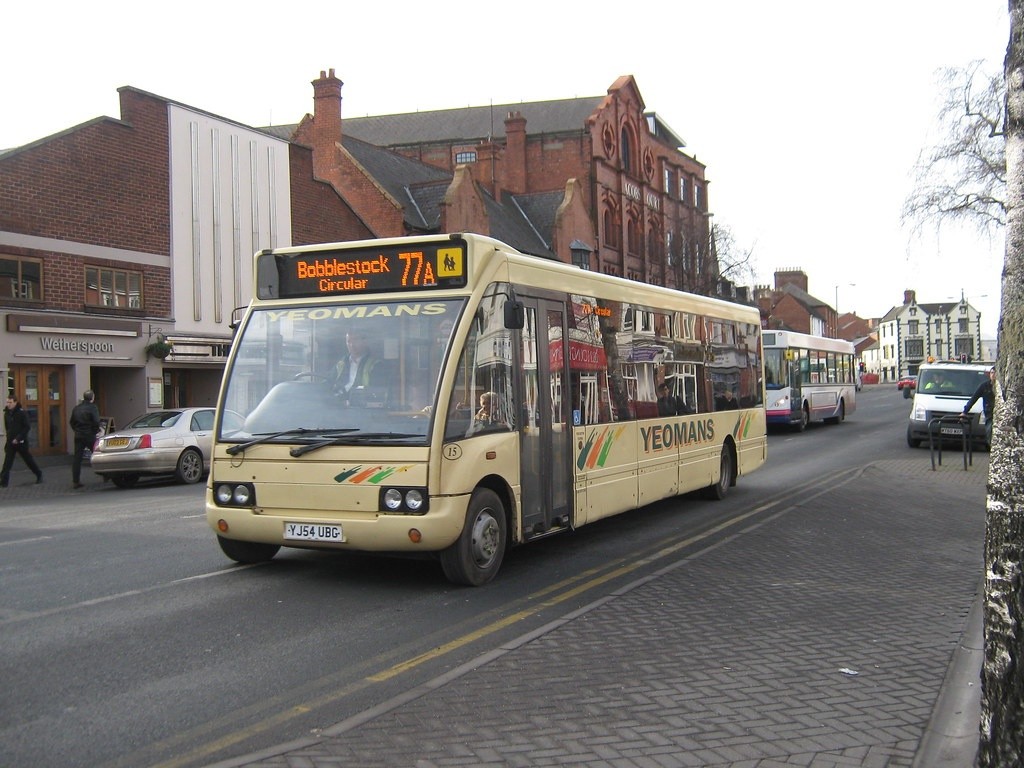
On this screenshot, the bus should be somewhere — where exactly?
[761,329,857,433]
[204,231,768,589]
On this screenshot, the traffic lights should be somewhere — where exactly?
[961,353,968,363]
[860,362,864,372]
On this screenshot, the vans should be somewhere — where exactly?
[907,363,995,453]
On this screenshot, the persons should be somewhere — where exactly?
[961,367,997,448]
[655,383,691,418]
[925,372,958,390]
[476,391,509,423]
[0,396,45,487]
[326,330,385,403]
[69,389,108,488]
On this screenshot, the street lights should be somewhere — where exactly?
[948,296,970,360]
[835,283,856,340]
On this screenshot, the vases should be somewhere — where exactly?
[152,347,170,357]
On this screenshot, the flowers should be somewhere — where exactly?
[149,336,174,353]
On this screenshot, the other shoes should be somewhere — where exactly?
[36,474,43,484]
[74,483,86,488]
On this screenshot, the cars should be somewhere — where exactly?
[90,406,247,488]
[898,376,917,391]
